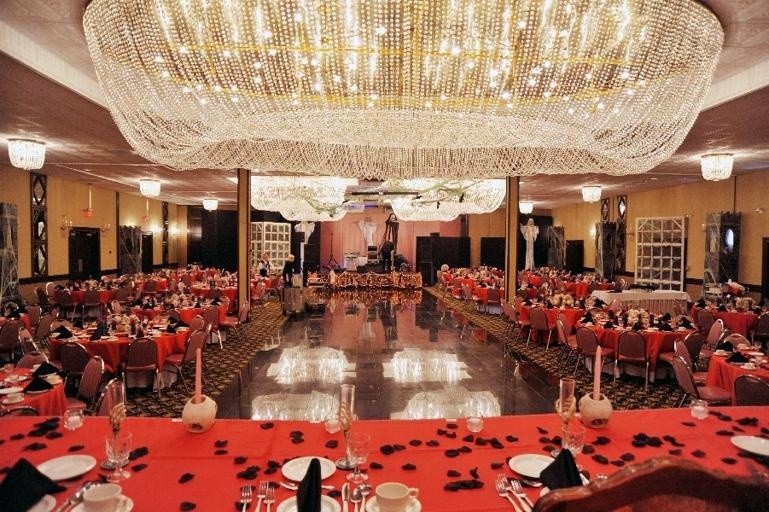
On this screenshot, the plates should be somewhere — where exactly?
[509,453,556,478]
[365,492,422,512]
[0,361,61,404]
[26,494,56,512]
[52,318,189,343]
[71,495,134,512]
[127,296,212,311]
[730,435,769,458]
[281,455,338,481]
[276,494,341,512]
[580,312,688,332]
[36,454,97,482]
[713,342,768,370]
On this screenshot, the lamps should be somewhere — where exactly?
[582,186,603,205]
[202,197,218,212]
[248,174,506,214]
[81,1,727,178]
[139,179,161,199]
[280,192,459,223]
[6,139,47,171]
[249,338,502,423]
[519,201,533,214]
[700,153,733,182]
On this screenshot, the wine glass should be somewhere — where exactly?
[551,378,576,459]
[334,384,360,469]
[100,382,130,471]
[344,434,370,483]
[103,430,131,482]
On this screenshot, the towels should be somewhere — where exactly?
[0,458,67,511]
[295,458,322,512]
[538,447,582,490]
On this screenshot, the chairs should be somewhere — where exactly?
[528,453,768,512]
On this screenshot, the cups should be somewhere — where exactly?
[83,482,128,512]
[689,399,708,419]
[375,481,419,512]
[560,306,565,310]
[560,425,586,454]
[63,410,84,431]
[467,417,484,432]
[325,416,341,433]
[731,309,737,314]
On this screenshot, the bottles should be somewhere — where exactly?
[579,393,613,428]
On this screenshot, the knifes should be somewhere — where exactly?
[57,480,99,512]
[341,481,350,511]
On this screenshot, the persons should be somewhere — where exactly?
[282,250,297,286]
[353,321,378,346]
[520,218,541,270]
[258,253,271,278]
[356,215,381,255]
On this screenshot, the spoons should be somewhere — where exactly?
[523,479,542,486]
[279,479,334,490]
[349,484,373,511]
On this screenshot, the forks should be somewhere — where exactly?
[495,473,536,512]
[239,478,274,511]
